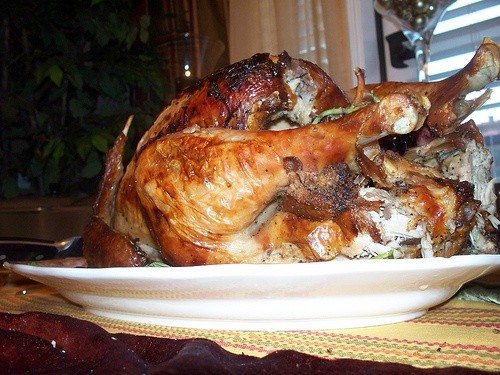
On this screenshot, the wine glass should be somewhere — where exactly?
[374,0,458,82]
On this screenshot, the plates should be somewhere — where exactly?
[4,253,500,332]
